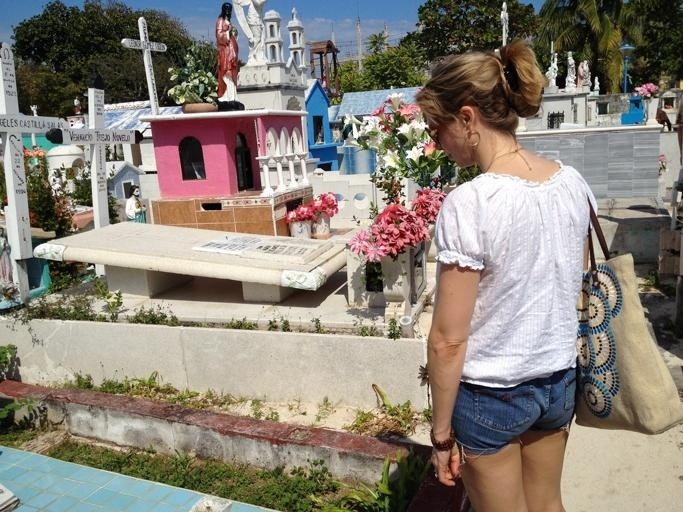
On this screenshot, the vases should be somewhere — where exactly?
[288,213,331,239]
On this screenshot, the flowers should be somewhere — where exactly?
[346,85,451,263]
[283,192,340,223]
[634,83,659,100]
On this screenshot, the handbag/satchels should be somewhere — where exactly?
[574,192,682,434]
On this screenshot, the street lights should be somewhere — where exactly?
[618,43,637,94]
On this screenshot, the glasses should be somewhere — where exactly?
[425,123,443,145]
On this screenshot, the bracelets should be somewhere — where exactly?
[430,426,456,450]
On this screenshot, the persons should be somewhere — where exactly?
[0,226,13,282]
[215,2,241,102]
[414,40,596,512]
[125,185,147,223]
